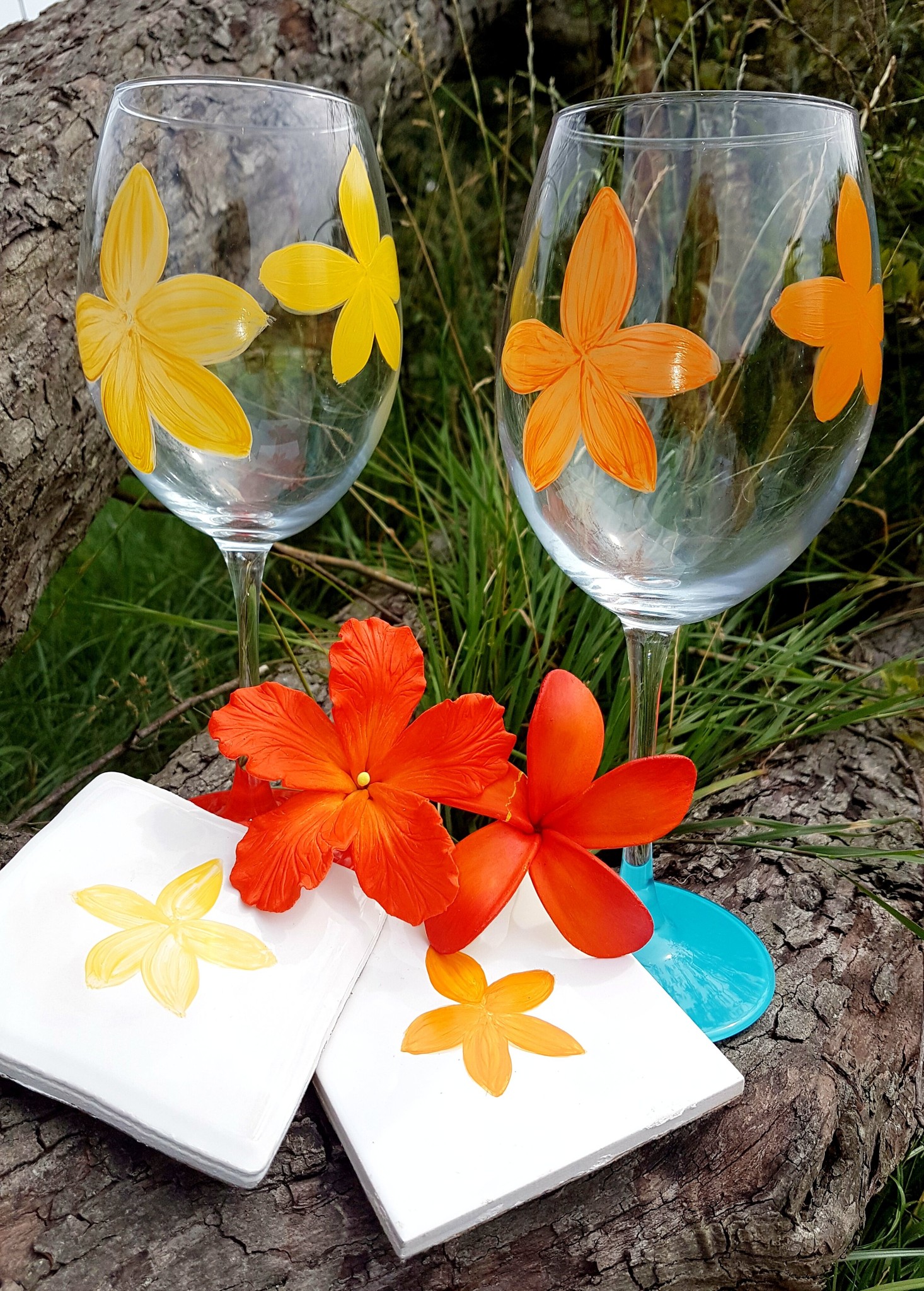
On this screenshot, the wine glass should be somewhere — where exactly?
[74,74,404,823]
[497,89,885,1040]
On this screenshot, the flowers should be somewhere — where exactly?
[190,615,698,957]
